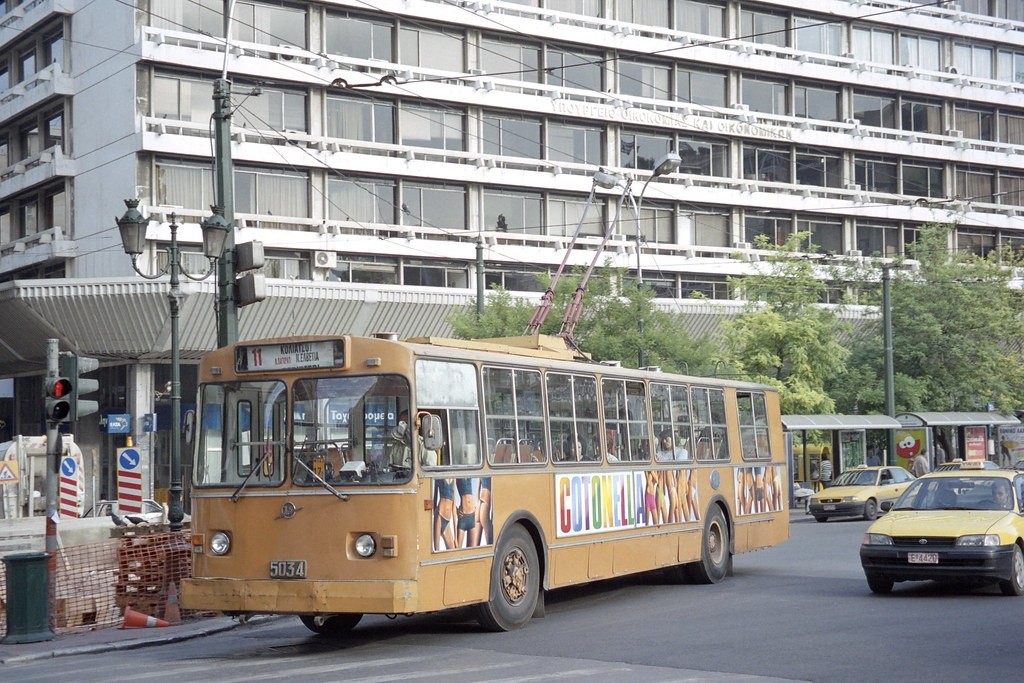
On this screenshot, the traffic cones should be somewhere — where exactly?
[164,582,184,626]
[122,605,170,631]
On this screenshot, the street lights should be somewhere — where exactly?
[115,197,231,580]
[592,152,683,368]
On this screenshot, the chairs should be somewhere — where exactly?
[931,490,957,507]
[707,437,722,461]
[697,437,710,459]
[537,435,660,462]
[491,438,515,463]
[685,434,699,459]
[488,438,497,460]
[514,439,533,463]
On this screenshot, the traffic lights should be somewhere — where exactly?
[44,377,73,423]
[59,351,99,421]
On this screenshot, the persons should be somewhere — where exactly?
[936,444,946,467]
[655,430,689,460]
[1000,435,1013,467]
[433,478,493,550]
[644,469,700,525]
[819,452,833,489]
[589,438,618,461]
[560,434,595,462]
[991,480,1012,509]
[912,448,930,477]
[736,466,782,515]
[866,450,880,466]
[382,409,437,483]
[698,413,727,442]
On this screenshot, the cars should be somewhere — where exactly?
[859,460,1024,595]
[805,464,924,523]
[934,458,1000,471]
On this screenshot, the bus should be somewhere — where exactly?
[175,332,792,638]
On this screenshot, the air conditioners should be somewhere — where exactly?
[844,118,860,131]
[312,251,337,269]
[841,53,856,65]
[732,104,749,115]
[610,234,626,242]
[946,130,964,144]
[469,69,487,83]
[845,183,861,196]
[582,235,605,251]
[733,242,751,249]
[365,58,389,76]
[944,4,962,17]
[277,43,302,63]
[944,65,960,81]
[904,63,917,74]
[279,129,309,148]
[846,250,863,264]
